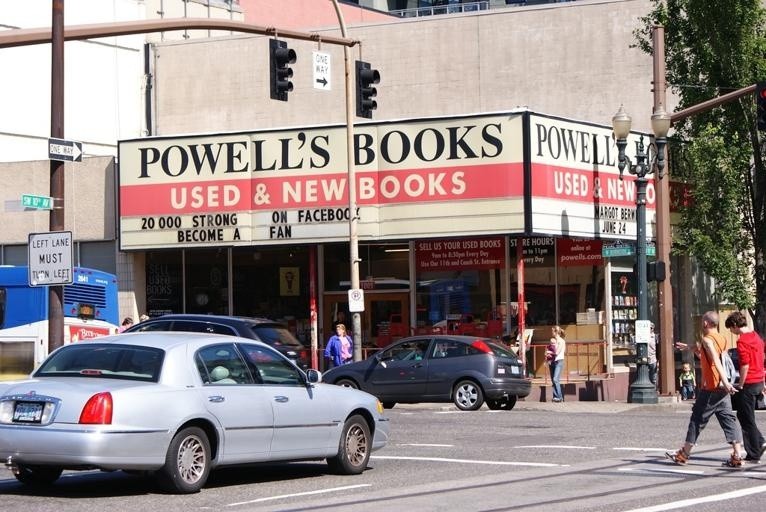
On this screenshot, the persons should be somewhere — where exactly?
[679,362,696,400]
[544,337,556,366]
[647,322,657,387]
[414,343,427,361]
[116,317,134,333]
[139,314,150,331]
[548,325,566,403]
[332,311,350,334]
[665,310,742,468]
[725,311,766,462]
[324,323,353,365]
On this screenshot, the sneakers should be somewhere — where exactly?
[744,443,766,464]
[721,454,743,468]
[665,449,688,465]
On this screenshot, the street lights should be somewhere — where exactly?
[612,104,670,404]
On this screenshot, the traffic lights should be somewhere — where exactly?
[269,39,296,102]
[354,61,380,119]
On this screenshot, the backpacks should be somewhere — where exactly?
[702,334,736,389]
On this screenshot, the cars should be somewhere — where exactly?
[319,334,531,411]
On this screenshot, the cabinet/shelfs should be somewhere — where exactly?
[612,293,638,346]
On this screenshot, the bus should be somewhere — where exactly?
[0,264,120,381]
